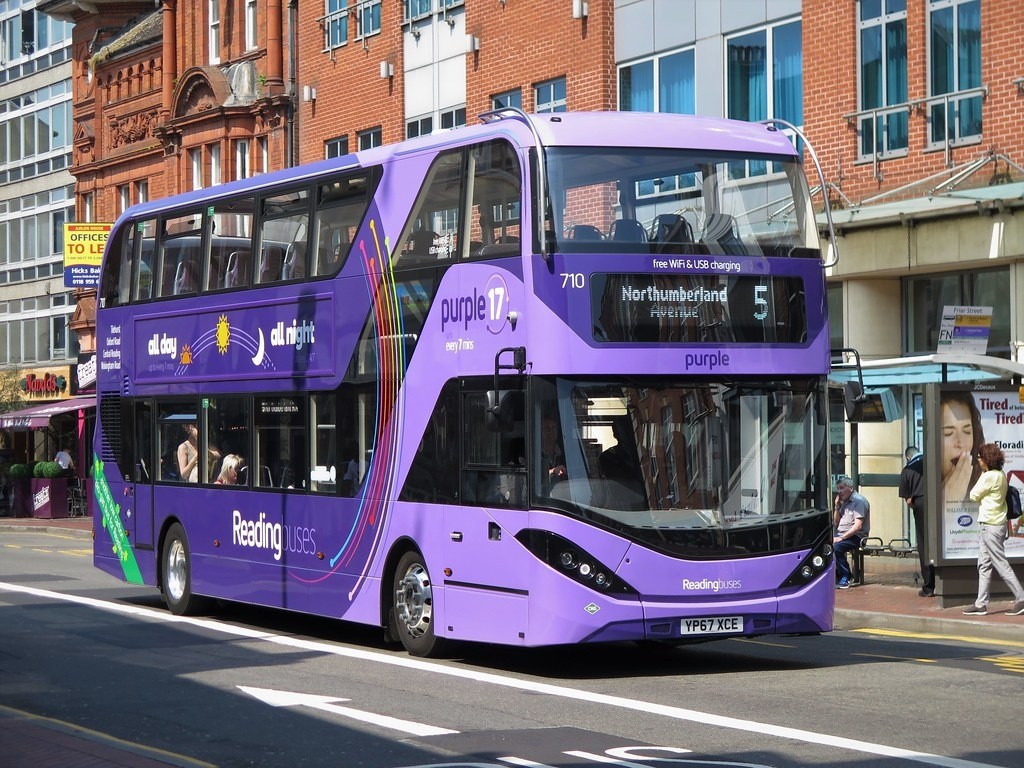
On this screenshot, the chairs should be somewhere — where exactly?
[148,213,740,301]
[210,459,296,489]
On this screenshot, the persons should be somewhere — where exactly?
[342,445,369,497]
[177,422,223,482]
[213,454,244,485]
[599,424,651,487]
[899,447,935,597]
[963,443,1024,616]
[939,392,985,511]
[541,417,566,480]
[54,446,75,469]
[833,477,870,588]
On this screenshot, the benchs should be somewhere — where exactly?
[846,536,921,590]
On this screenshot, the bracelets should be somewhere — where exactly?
[841,536,844,540]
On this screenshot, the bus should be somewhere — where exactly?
[85,105,868,661]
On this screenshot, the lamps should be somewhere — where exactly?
[380,61,393,78]
[464,34,479,52]
[572,0,588,18]
[304,86,316,101]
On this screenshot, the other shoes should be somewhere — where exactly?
[918,589,935,598]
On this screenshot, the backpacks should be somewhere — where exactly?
[1006,485,1023,519]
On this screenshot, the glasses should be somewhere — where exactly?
[977,456,981,459]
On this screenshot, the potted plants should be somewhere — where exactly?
[86,465,93,516]
[9,461,69,520]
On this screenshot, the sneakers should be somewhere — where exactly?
[1005,601,1024,615]
[839,576,854,589]
[962,604,988,615]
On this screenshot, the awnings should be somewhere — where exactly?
[0,398,97,432]
